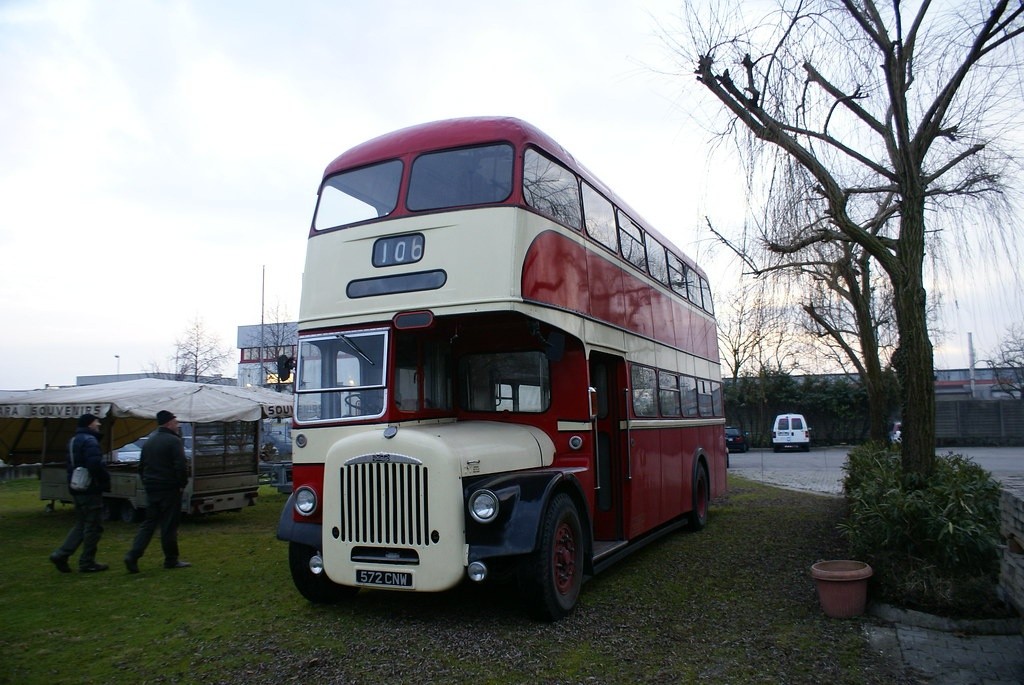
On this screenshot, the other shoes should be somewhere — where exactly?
[50,554,72,573]
[124,556,139,574]
[164,559,192,568]
[80,562,108,573]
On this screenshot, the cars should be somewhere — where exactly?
[886,421,902,449]
[259,432,292,457]
[726,427,752,453]
[112,435,280,459]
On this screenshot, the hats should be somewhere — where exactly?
[156,410,176,425]
[78,414,97,429]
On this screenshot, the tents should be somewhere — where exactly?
[0,378,321,468]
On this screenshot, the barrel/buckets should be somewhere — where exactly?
[810,560,873,618]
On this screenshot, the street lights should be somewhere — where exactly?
[116,355,120,376]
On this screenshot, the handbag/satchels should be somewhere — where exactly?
[70,467,91,489]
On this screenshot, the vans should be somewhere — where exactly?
[772,414,812,450]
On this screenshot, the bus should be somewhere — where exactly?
[276,113,726,616]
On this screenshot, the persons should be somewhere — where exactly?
[124,409,191,575]
[49,415,111,574]
